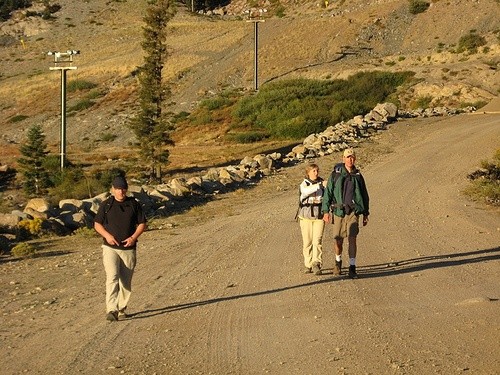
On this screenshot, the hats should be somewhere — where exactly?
[344,149,356,158]
[113,176,127,188]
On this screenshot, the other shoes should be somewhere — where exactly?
[305,268,312,274]
[118,313,127,320]
[312,262,322,275]
[106,312,118,321]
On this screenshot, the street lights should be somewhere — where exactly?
[244,8,268,91]
[47,50,80,172]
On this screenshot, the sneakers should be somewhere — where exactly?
[332,260,342,276]
[348,265,358,279]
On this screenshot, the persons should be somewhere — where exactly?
[321,148,371,279]
[93,176,148,322]
[298,164,327,275]
